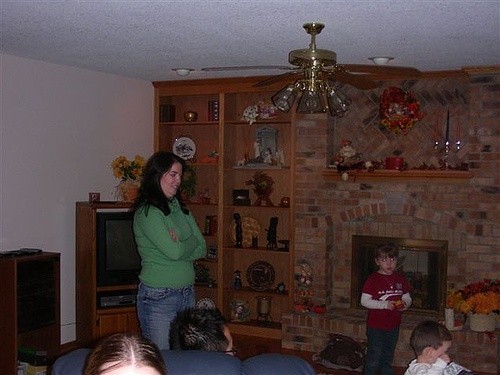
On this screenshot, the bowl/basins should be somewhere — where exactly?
[184,111,199,121]
[387,156,405,171]
[314,305,326,313]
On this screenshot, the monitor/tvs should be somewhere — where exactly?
[96,212,142,285]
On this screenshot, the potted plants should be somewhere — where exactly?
[384,150,404,170]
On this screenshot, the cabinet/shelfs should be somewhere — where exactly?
[151,76,294,340]
[75,201,142,348]
[0,251,62,375]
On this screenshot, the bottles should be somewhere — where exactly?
[233,268,242,290]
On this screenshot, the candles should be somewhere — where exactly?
[457,122,460,140]
[438,113,441,137]
[435,121,439,141]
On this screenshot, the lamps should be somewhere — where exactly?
[272,80,351,118]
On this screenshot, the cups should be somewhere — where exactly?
[209,100,218,122]
[255,295,271,317]
[159,104,175,123]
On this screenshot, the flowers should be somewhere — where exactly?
[366,87,425,138]
[448,278,500,315]
[110,153,147,182]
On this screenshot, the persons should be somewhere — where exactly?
[360,247,413,375]
[85,332,170,375]
[404,321,453,375]
[167,307,239,357]
[133,151,207,351]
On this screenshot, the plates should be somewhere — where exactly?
[247,261,275,292]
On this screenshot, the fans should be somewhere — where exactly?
[200,23,422,90]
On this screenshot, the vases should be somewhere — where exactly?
[119,177,142,201]
[470,312,497,331]
